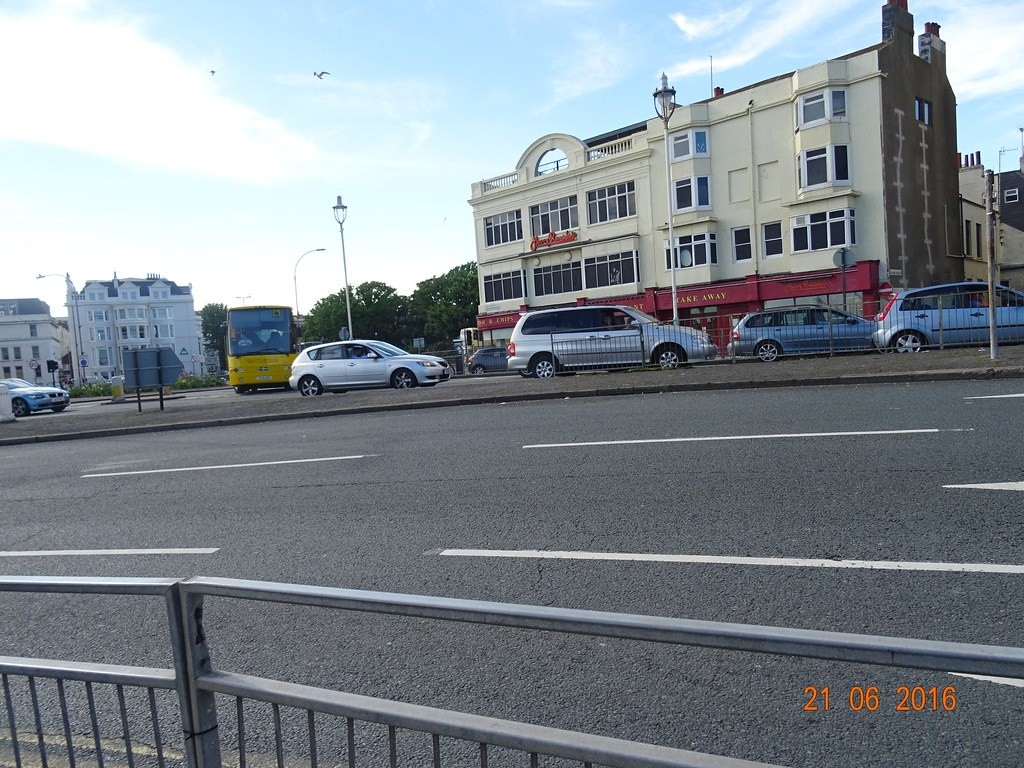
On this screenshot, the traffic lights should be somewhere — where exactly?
[51,360,58,370]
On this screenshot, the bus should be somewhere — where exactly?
[220,304,298,394]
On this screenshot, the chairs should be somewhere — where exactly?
[764,316,783,326]
[951,296,961,308]
[576,318,591,327]
[266,332,288,346]
[804,316,816,325]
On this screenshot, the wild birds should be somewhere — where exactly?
[314,71,330,79]
[211,71,215,76]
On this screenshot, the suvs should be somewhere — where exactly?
[506,306,718,380]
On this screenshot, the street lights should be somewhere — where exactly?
[332,194,354,341]
[35,273,86,386]
[293,248,326,353]
[651,71,678,326]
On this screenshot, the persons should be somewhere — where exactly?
[970,291,986,307]
[814,312,829,324]
[239,334,253,346]
[605,315,630,329]
[352,347,367,359]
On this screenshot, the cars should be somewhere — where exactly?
[0,377,71,418]
[466,346,509,375]
[726,304,876,363]
[872,282,1024,355]
[288,339,452,397]
[216,370,229,381]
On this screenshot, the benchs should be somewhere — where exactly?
[911,303,931,310]
